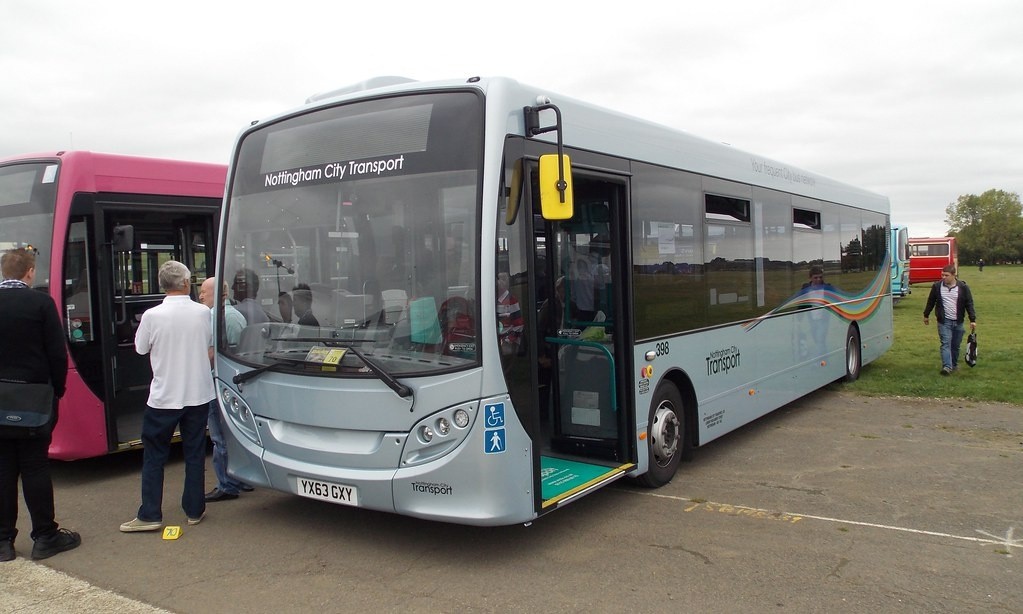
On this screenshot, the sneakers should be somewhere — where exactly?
[0,538,16,562]
[118,518,162,531]
[33,527,80,559]
[187,511,206,525]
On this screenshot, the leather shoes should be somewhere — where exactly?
[204,486,239,502]
[241,487,254,491]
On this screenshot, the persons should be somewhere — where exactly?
[283,284,320,349]
[497,272,523,351]
[569,255,595,322]
[276,292,300,350]
[118,261,217,531]
[978,259,984,271]
[540,276,579,359]
[923,265,976,374]
[0,249,81,562]
[226,268,269,365]
[796,265,837,366]
[200,277,254,501]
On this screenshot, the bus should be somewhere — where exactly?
[1,148,310,461]
[908,235,960,285]
[891,223,912,304]
[212,74,895,528]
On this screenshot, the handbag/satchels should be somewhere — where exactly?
[965,330,978,367]
[0,379,54,436]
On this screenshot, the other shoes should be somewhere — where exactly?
[941,367,953,375]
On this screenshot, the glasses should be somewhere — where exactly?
[277,300,290,307]
[811,276,823,279]
